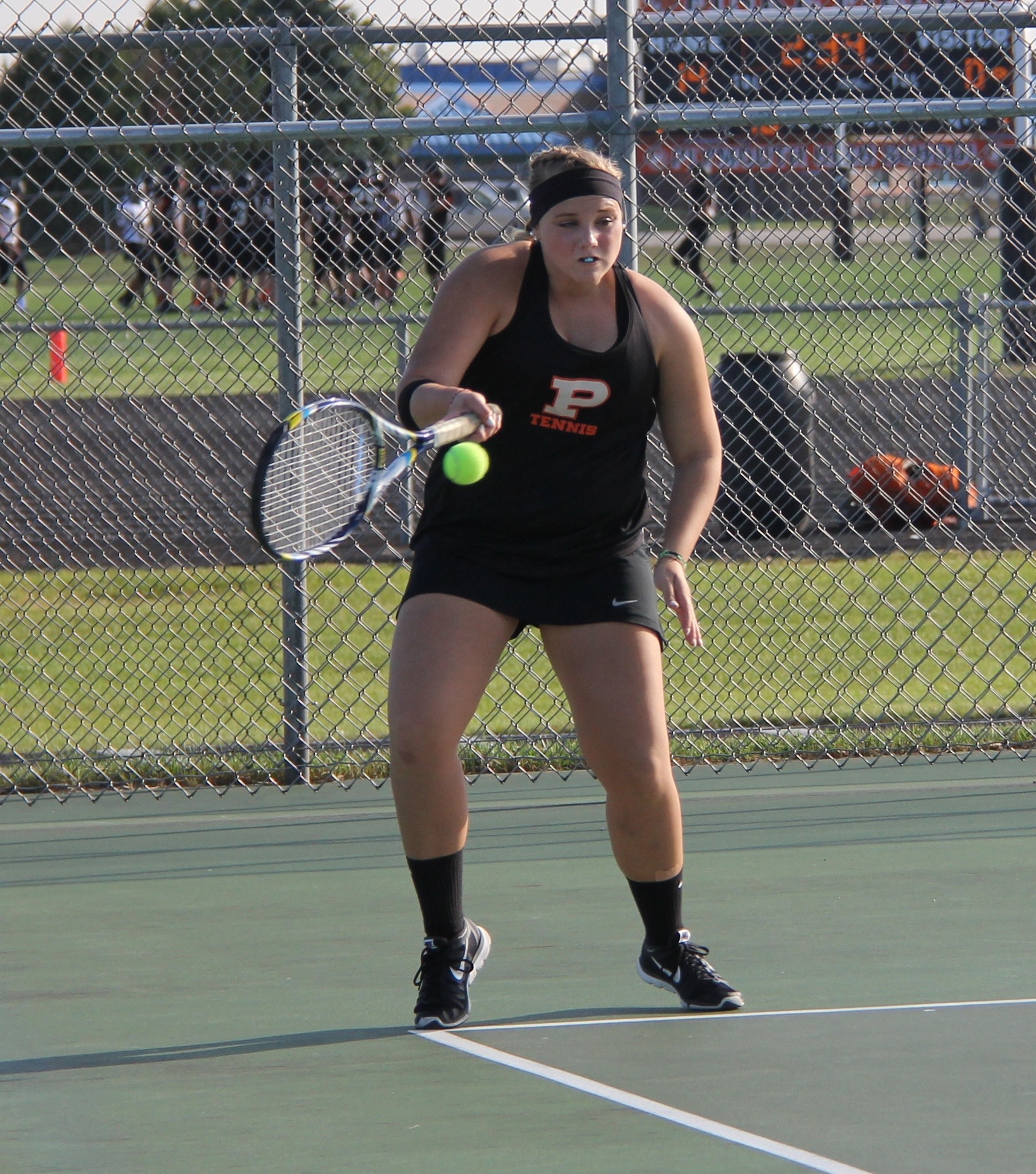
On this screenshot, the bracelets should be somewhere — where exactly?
[450,388,470,405]
[652,549,687,574]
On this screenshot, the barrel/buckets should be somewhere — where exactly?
[709,350,813,537]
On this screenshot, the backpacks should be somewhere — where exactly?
[849,454,977,530]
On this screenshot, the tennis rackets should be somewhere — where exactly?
[249,397,503,562]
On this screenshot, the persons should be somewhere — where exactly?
[116,149,455,315]
[384,146,744,1029]
[0,179,31,314]
[671,164,723,299]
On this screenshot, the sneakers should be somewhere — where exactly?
[637,930,745,1010]
[413,918,491,1027]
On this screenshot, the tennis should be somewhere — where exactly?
[441,440,490,486]
